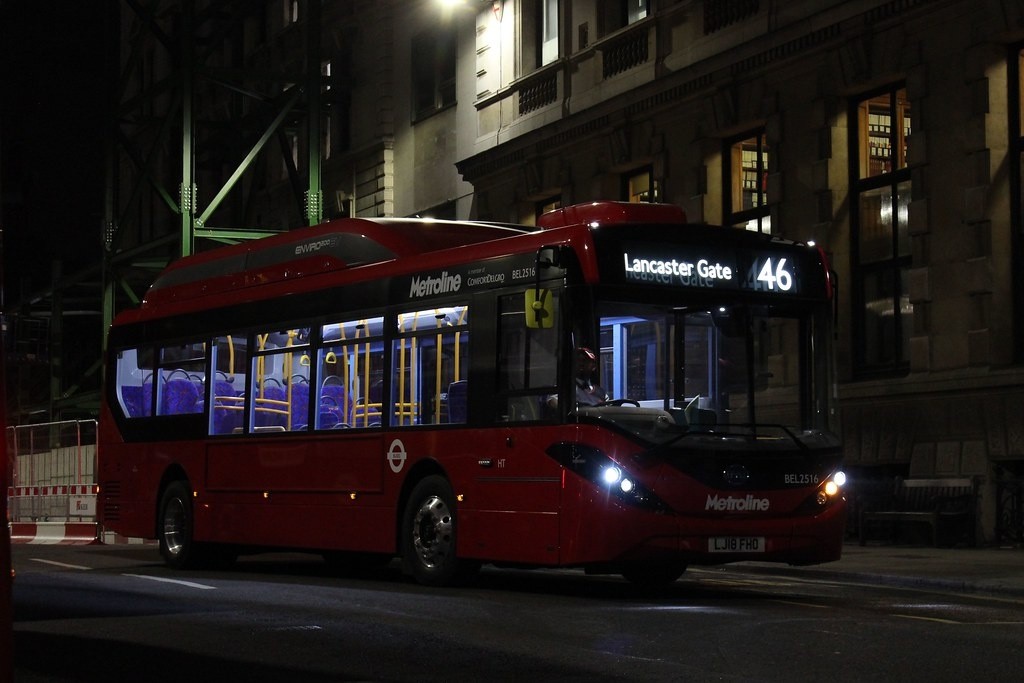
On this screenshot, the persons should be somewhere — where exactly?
[546,348,608,406]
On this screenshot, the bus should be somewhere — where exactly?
[96,198,852,590]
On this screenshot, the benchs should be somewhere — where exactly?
[858,476,977,548]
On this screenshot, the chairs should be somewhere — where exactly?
[138,369,383,434]
[448,379,468,423]
[543,350,616,422]
